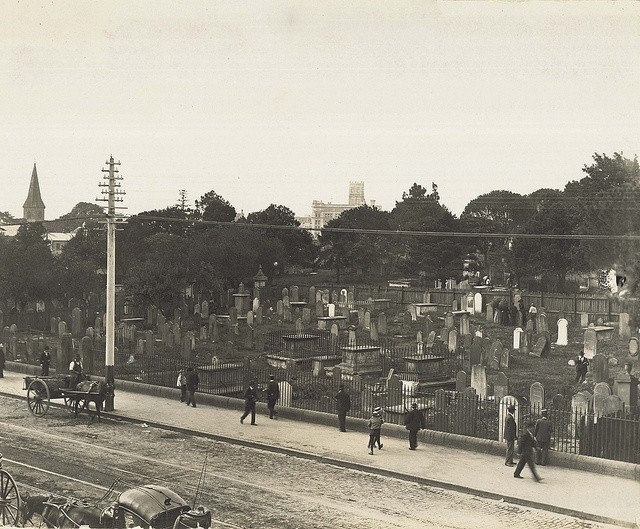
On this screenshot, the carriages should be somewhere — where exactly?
[18,453,211,529]
[23,372,115,424]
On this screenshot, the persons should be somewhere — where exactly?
[333,384,350,432]
[404,402,425,450]
[186,368,198,408]
[533,409,555,466]
[367,411,384,455]
[490,298,538,327]
[574,351,589,383]
[40,346,51,376]
[266,375,280,419]
[69,354,84,390]
[176,368,187,403]
[503,405,517,467]
[0,343,6,377]
[513,422,544,482]
[240,381,258,426]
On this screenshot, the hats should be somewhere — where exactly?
[506,406,516,411]
[411,403,418,408]
[248,380,256,387]
[372,407,382,414]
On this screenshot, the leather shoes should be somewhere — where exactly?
[515,474,523,478]
[378,443,383,450]
[506,463,513,466]
